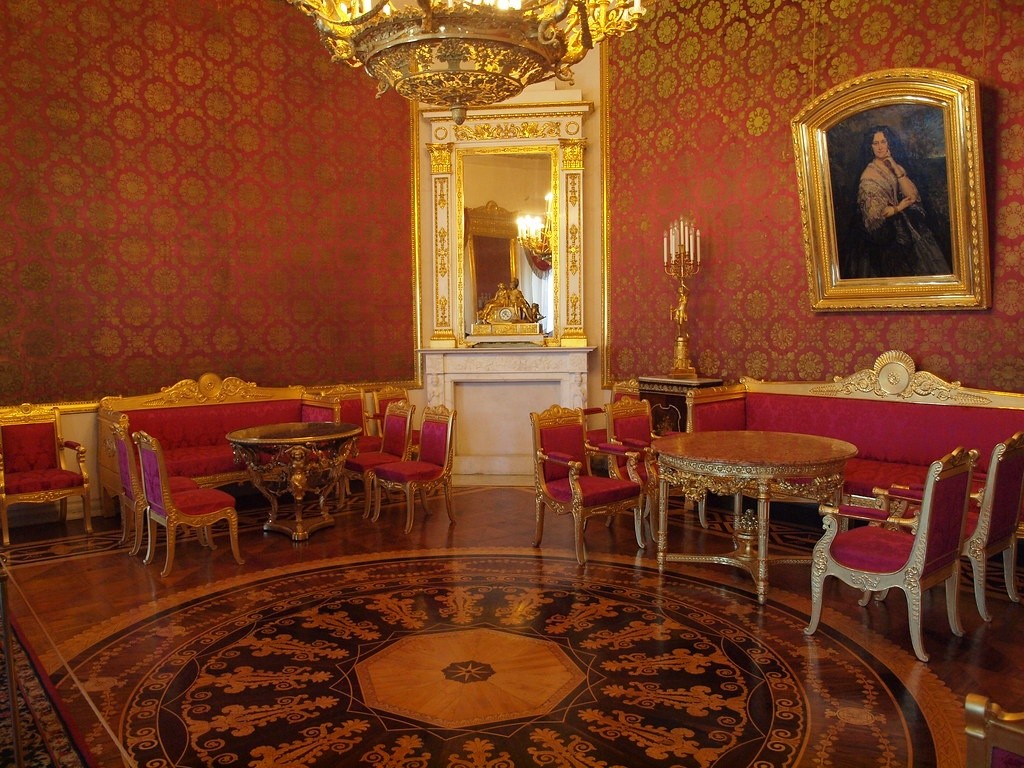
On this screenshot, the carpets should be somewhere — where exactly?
[0,611,100,768]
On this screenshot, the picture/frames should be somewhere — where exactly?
[790,68,992,312]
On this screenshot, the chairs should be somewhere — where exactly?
[320,384,377,496]
[0,403,93,547]
[964,693,1024,767]
[109,423,209,556]
[341,400,416,519]
[372,386,420,453]
[803,446,980,663]
[874,431,1024,622]
[529,404,647,566]
[131,430,246,577]
[370,405,457,534]
[603,396,709,543]
[583,379,640,456]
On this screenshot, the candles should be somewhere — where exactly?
[663,214,701,267]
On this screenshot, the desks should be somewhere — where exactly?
[651,430,858,606]
[225,422,362,541]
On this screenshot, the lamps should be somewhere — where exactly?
[517,192,552,266]
[289,0,647,126]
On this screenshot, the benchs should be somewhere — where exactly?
[97,373,341,531]
[685,350,1024,539]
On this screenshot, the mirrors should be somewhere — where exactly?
[456,144,560,348]
